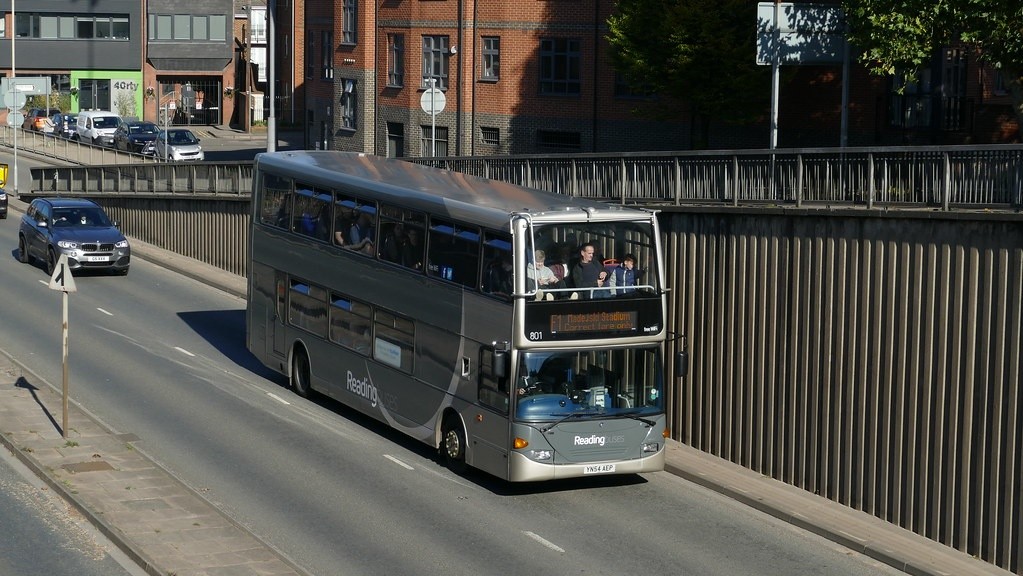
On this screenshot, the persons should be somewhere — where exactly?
[290,189,647,301]
[77,211,94,226]
[504,356,543,408]
[50,213,66,225]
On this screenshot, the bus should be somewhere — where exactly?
[245,150,688,483]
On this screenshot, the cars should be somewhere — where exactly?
[0,179,8,219]
[113,121,161,155]
[51,112,78,138]
[155,128,204,162]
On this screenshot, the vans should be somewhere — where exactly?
[76,111,123,147]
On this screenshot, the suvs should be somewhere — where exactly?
[21,108,62,132]
[19,196,131,276]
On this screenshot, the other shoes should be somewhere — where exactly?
[535,289,543,301]
[570,292,578,299]
[547,293,553,300]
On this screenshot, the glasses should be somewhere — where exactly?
[397,227,399,229]
